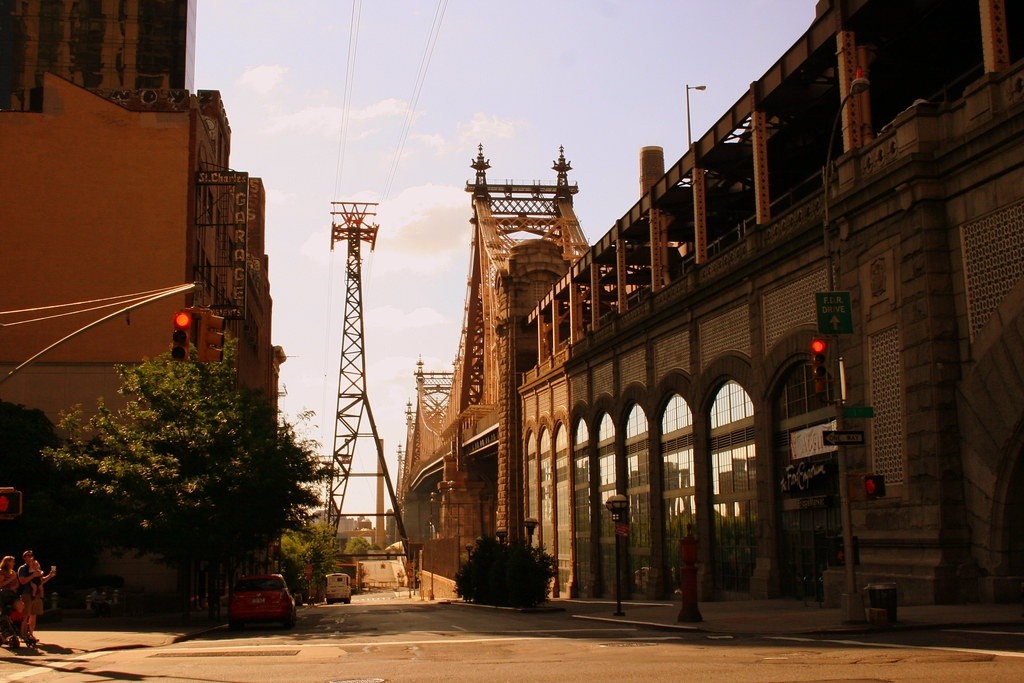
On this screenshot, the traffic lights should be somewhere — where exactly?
[171,313,193,362]
[861,474,887,498]
[0,488,23,521]
[198,311,225,363]
[812,338,831,390]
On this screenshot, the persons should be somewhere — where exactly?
[0,550,56,645]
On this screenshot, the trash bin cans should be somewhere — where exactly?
[864,582,897,623]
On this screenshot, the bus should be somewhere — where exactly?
[335,562,361,592]
[325,573,352,604]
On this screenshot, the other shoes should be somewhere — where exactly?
[31,595,35,600]
[30,635,39,642]
[40,597,45,600]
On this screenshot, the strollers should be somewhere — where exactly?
[0,601,36,650]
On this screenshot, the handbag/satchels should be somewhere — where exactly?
[0,588,16,611]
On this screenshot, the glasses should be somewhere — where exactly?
[26,554,34,556]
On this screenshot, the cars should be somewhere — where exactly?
[227,574,298,629]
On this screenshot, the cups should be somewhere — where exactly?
[51,566,56,575]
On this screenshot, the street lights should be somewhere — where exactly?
[606,495,630,617]
[524,518,539,546]
[386,534,412,599]
[496,526,507,544]
[823,78,874,620]
[466,543,473,559]
[686,86,706,149]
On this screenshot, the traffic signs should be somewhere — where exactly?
[822,431,865,446]
